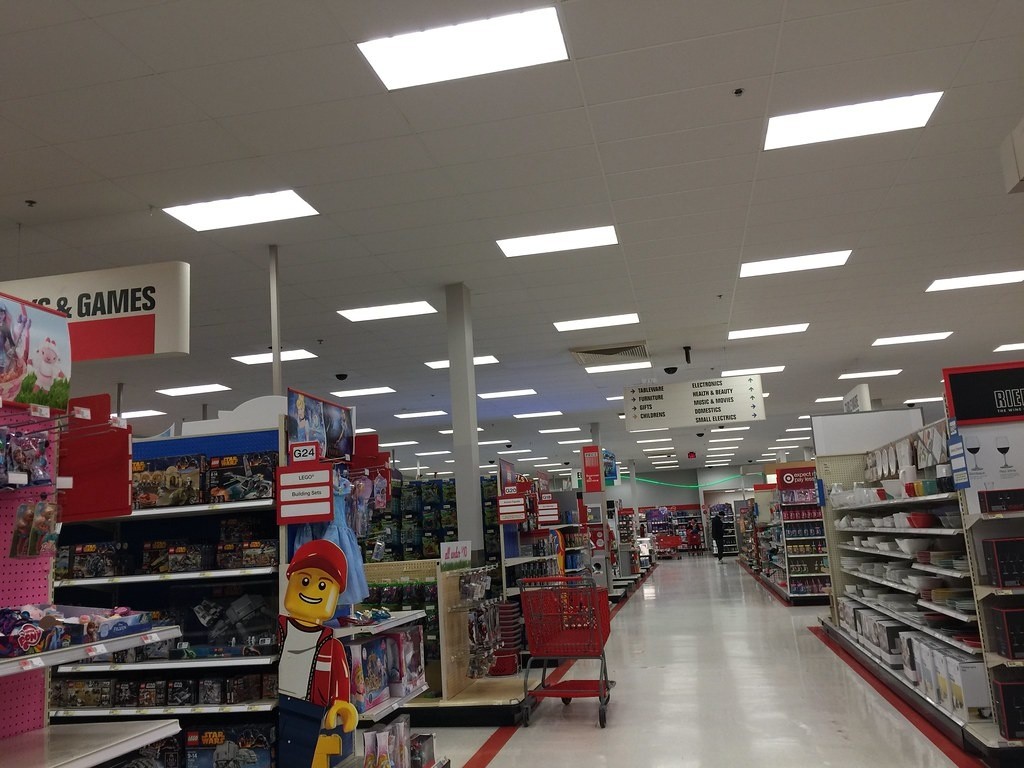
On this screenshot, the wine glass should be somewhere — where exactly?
[964,433,984,474]
[995,434,1015,472]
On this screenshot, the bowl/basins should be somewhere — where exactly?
[849,535,933,556]
[852,510,963,530]
[902,573,944,589]
[862,588,888,599]
[859,479,901,503]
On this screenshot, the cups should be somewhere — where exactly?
[905,465,958,496]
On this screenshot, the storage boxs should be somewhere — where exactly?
[47,449,436,768]
[836,596,993,724]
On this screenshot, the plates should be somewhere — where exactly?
[862,438,910,481]
[939,624,982,649]
[841,555,858,569]
[876,594,924,623]
[916,550,970,573]
[921,586,976,613]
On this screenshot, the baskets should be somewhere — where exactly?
[490,602,522,676]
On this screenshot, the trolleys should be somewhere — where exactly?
[516,576,616,728]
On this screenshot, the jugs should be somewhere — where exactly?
[899,464,916,499]
[829,481,846,508]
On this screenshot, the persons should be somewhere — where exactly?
[686,518,700,556]
[712,511,728,564]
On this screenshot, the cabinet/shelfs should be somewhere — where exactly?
[50,388,458,768]
[810,361,1024,768]
[0,291,183,768]
[504,387,831,743]
